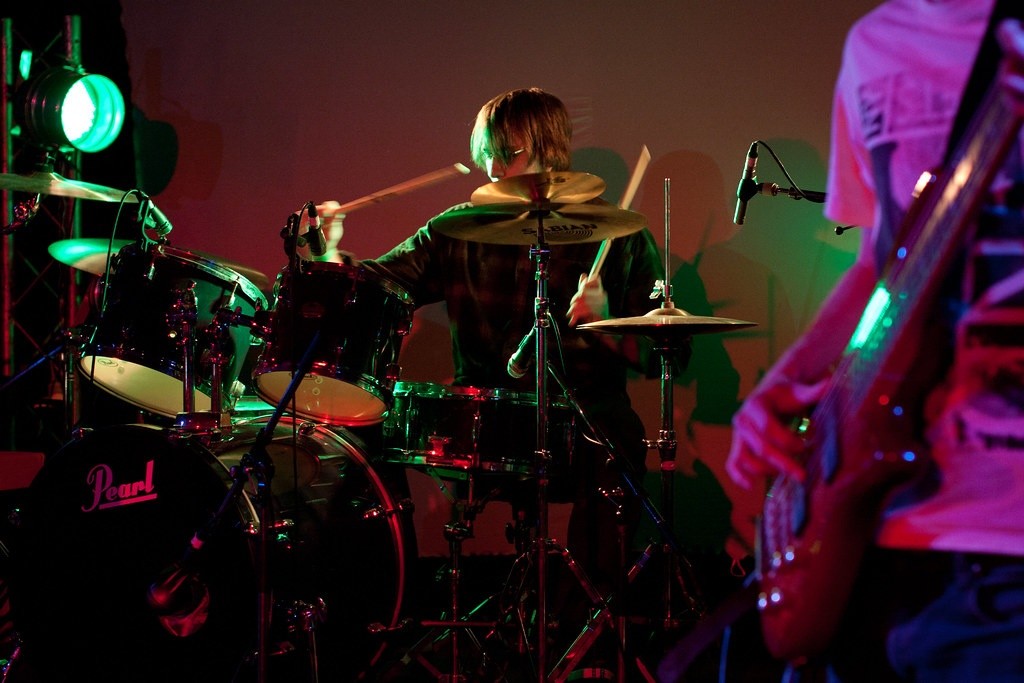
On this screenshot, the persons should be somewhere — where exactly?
[725,0,1024,683]
[269,87,690,612]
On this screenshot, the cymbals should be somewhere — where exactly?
[576,307,760,334]
[429,172,650,247]
[47,235,271,295]
[0,170,141,204]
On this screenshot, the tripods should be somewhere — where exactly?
[379,214,709,683]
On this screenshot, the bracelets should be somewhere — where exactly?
[313,247,337,262]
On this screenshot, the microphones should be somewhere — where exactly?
[507,328,536,378]
[147,583,178,608]
[136,191,173,237]
[308,202,328,255]
[734,142,758,226]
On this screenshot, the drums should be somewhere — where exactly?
[385,378,580,483]
[7,411,420,682]
[74,241,269,422]
[250,258,418,428]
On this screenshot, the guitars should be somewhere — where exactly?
[751,54,1022,663]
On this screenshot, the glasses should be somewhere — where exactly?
[483,147,527,168]
